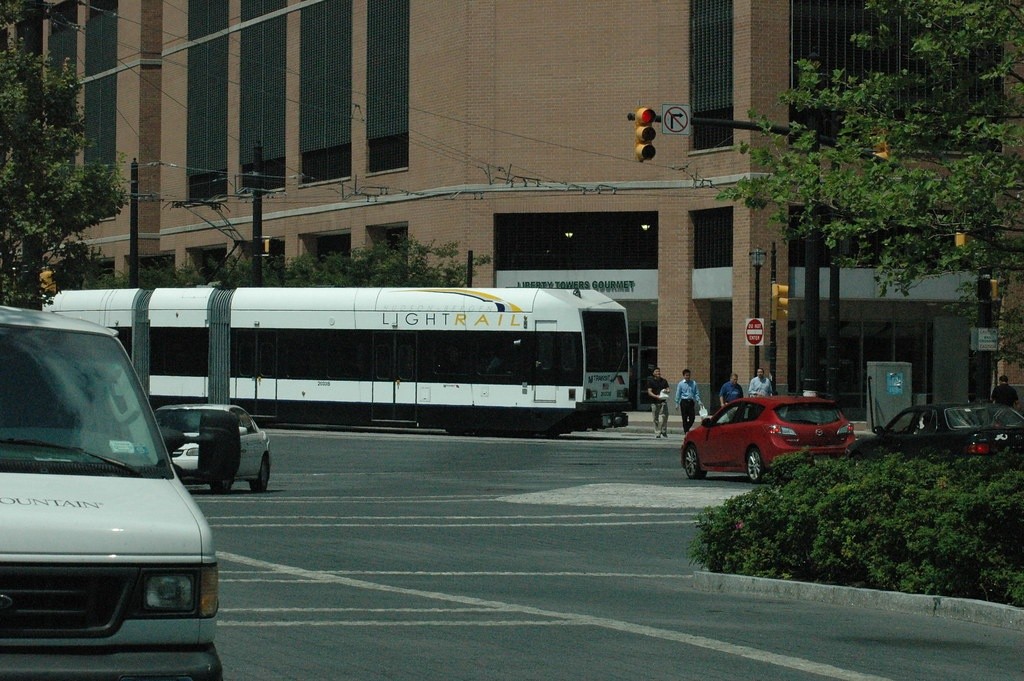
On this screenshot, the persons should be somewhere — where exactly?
[717,374,744,422]
[646,368,671,438]
[674,369,704,434]
[989,376,1021,412]
[747,369,774,398]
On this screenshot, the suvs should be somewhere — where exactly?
[0,305,225,681]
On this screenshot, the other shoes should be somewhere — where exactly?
[656,436,660,438]
[661,432,668,438]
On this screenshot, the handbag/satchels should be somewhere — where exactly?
[699,405,708,420]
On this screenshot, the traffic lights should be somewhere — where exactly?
[39,270,66,294]
[978,279,998,302]
[635,109,656,163]
[264,238,283,255]
[772,282,790,323]
[871,123,890,163]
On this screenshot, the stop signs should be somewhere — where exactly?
[744,316,763,347]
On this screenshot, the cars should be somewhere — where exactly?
[153,405,272,492]
[854,401,1023,459]
[680,397,855,482]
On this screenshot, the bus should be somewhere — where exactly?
[43,288,631,440]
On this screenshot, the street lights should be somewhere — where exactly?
[467,258,497,288]
[750,245,767,378]
